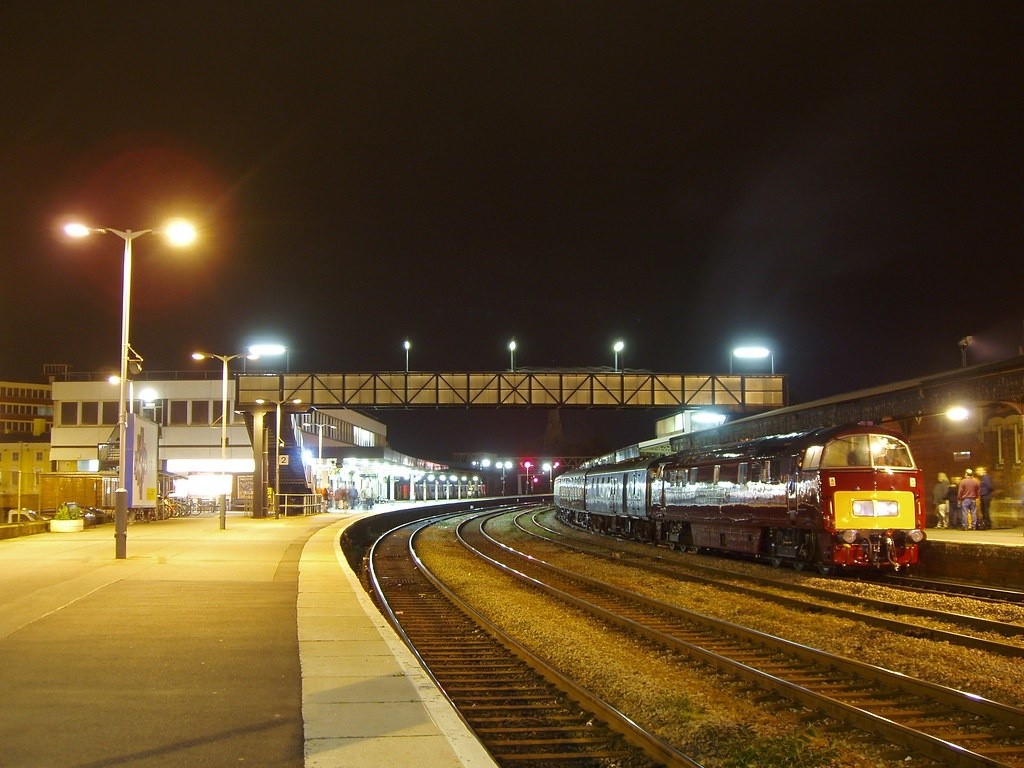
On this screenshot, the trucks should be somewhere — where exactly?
[8,510,39,523]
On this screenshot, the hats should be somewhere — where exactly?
[966,469,973,475]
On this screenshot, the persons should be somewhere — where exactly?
[980,467,994,530]
[348,486,358,509]
[933,473,950,528]
[958,469,980,531]
[364,486,373,510]
[875,446,902,466]
[949,468,983,529]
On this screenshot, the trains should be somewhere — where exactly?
[550,422,926,575]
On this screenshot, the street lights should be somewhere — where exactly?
[729,347,775,375]
[64,219,197,560]
[405,341,410,372]
[495,462,513,497]
[543,464,552,492]
[614,341,623,372]
[428,474,466,501]
[524,462,531,489]
[193,351,260,530]
[304,423,337,463]
[510,341,516,372]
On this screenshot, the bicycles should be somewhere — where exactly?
[109,497,203,523]
